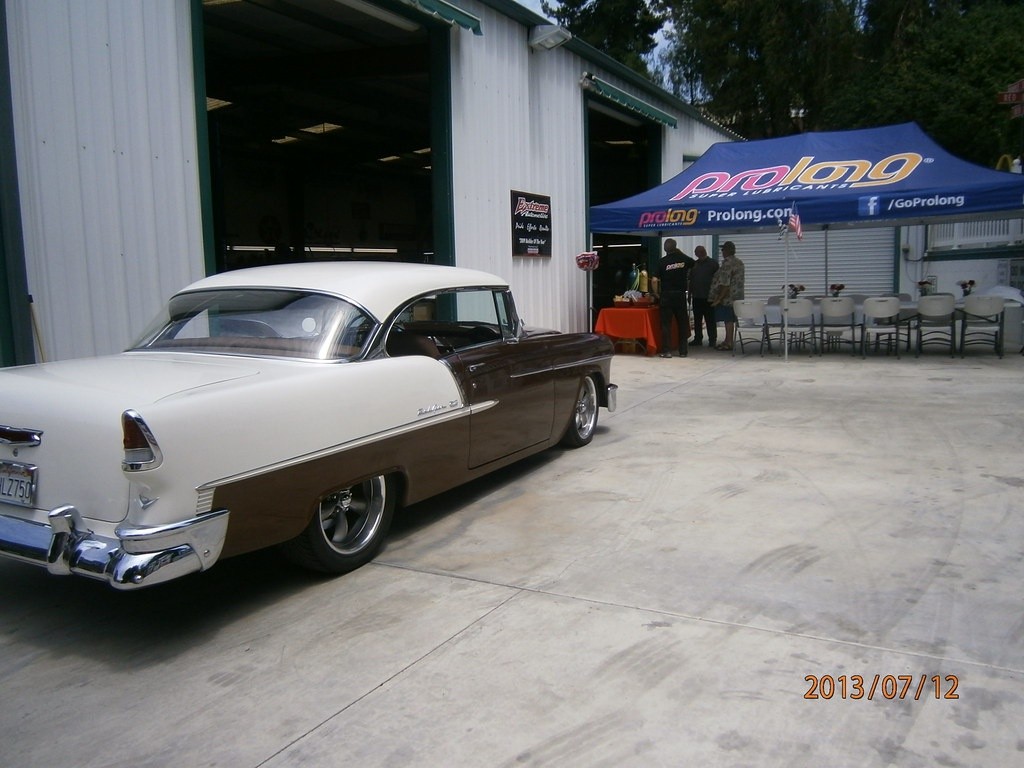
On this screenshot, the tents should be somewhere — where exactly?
[589,121,1024,360]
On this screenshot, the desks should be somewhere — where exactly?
[759,303,1021,351]
[595,305,692,356]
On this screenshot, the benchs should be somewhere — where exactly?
[395,324,479,352]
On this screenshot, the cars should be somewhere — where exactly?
[0,259,620,594]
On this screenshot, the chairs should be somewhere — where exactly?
[731,292,1006,359]
[387,336,440,360]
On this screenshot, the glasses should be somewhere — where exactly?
[721,248,724,252]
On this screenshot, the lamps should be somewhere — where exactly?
[530,23,572,52]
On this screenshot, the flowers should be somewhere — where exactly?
[945,279,975,295]
[916,281,932,295]
[829,284,844,298]
[782,284,805,298]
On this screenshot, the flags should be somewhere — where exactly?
[789,204,802,240]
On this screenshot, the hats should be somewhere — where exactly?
[718,241,736,254]
[664,239,676,251]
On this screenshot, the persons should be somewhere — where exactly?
[652,238,695,357]
[688,246,719,346]
[708,241,744,350]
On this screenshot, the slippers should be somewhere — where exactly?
[714,341,727,348]
[717,344,733,351]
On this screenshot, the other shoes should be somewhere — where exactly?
[689,338,703,346]
[657,352,672,358]
[708,338,716,346]
[679,351,687,357]
